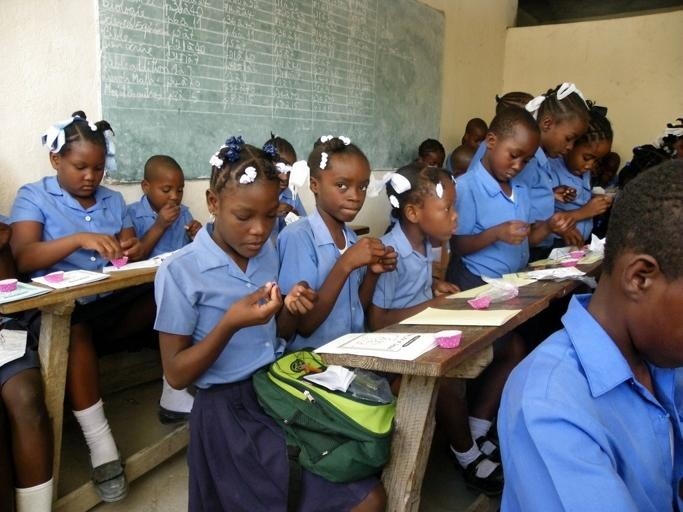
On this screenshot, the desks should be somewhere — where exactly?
[317,243,603,510]
[1,252,190,512]
[348,224,371,237]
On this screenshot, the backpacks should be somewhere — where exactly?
[253,347,397,482]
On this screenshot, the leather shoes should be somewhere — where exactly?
[90,460,129,502]
[460,437,504,496]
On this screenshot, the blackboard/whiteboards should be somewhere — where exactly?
[99,0,445,184]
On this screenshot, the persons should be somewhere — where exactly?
[126,155,205,258]
[154,134,386,512]
[449,81,624,294]
[6,111,196,503]
[263,134,523,491]
[1,217,53,512]
[496,108,683,511]
